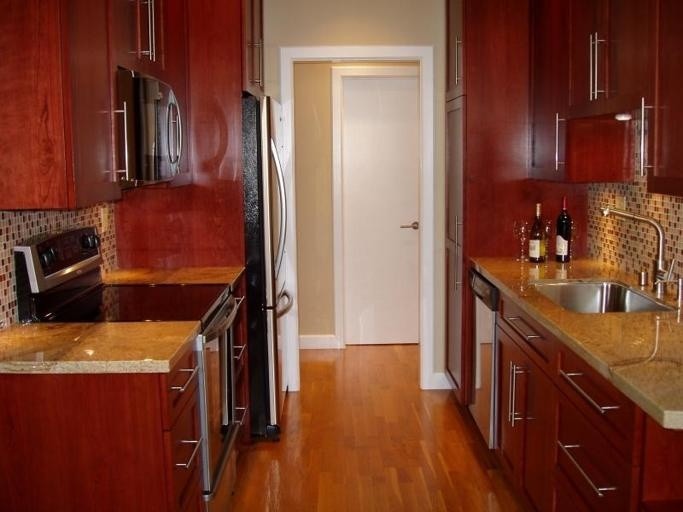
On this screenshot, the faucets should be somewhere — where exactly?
[600,204,675,295]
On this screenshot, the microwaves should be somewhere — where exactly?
[133,76,183,186]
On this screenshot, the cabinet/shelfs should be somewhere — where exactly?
[552,342,681,510]
[519,0,681,199]
[436,3,588,408]
[232,277,253,467]
[0,1,193,215]
[0,349,207,511]
[490,298,566,510]
[114,1,267,108]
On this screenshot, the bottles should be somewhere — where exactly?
[554,208,572,263]
[528,203,546,263]
[529,266,569,282]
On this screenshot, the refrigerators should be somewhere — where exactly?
[261,95,294,441]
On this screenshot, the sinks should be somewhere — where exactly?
[529,279,682,315]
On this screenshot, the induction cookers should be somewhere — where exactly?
[28,266,234,333]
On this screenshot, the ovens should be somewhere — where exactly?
[194,294,249,511]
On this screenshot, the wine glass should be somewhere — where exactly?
[511,219,576,265]
